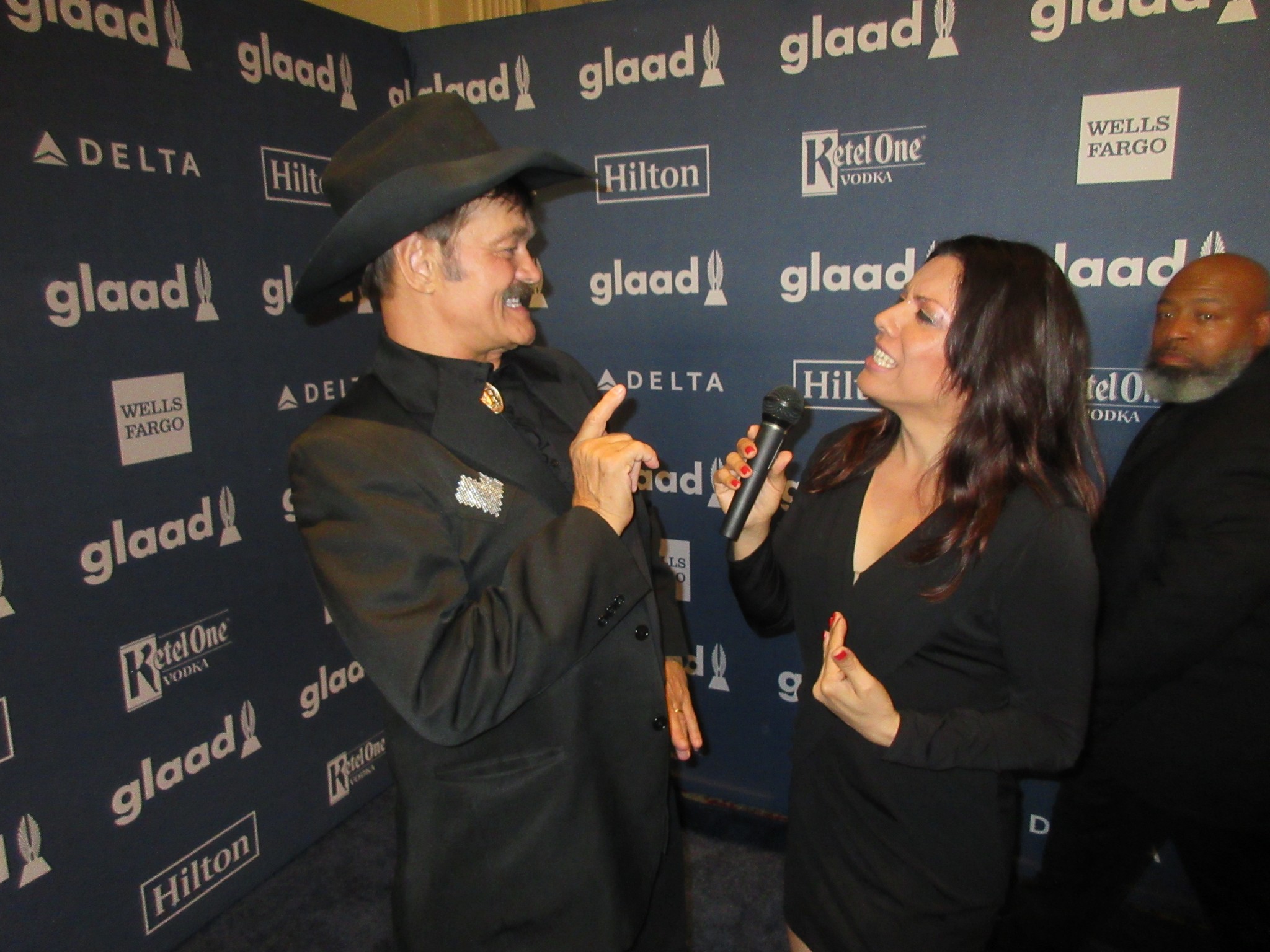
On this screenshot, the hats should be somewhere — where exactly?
[291,94,602,324]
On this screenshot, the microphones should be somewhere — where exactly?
[720,384,807,541]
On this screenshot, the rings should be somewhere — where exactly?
[675,708,684,714]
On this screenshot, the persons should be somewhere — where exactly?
[712,234,1108,952]
[1065,255,1269,952]
[285,92,706,952]
